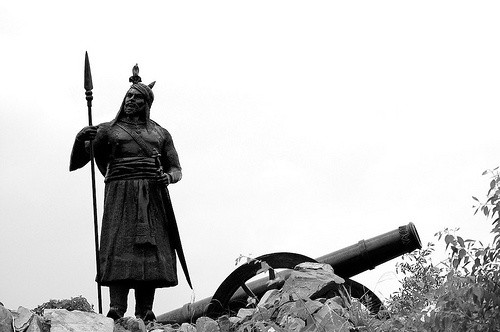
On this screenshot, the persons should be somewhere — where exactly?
[73,64,193,322]
